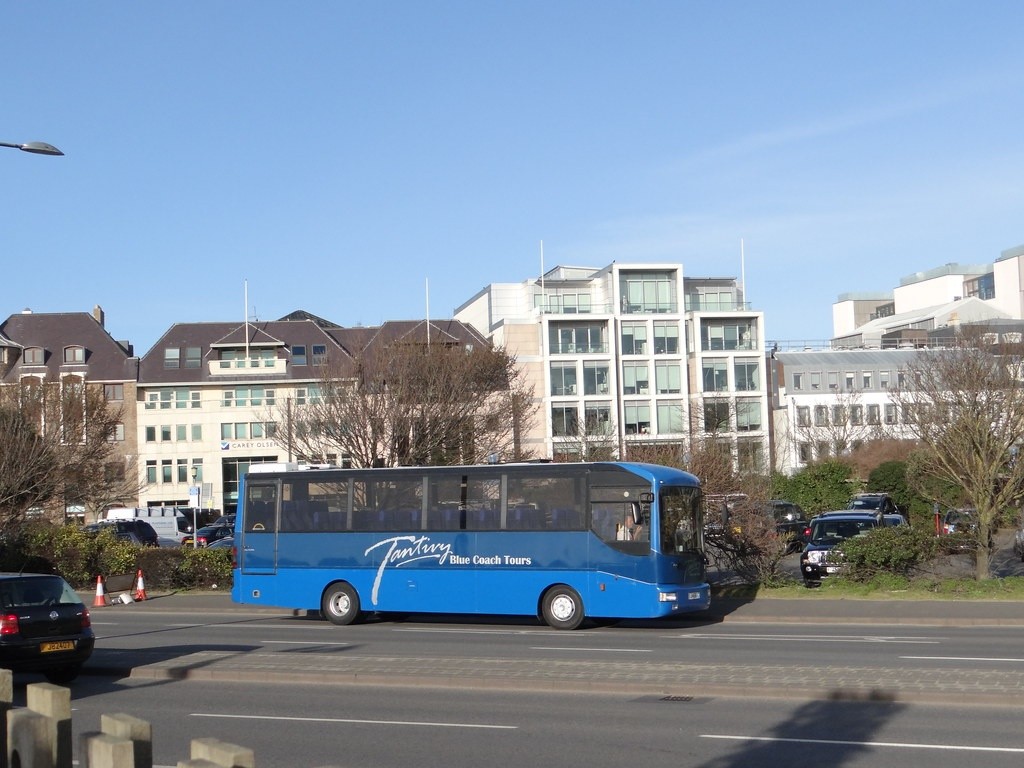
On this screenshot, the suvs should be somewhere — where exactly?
[799,509,891,588]
[703,501,810,557]
[83,518,161,549]
[847,492,899,515]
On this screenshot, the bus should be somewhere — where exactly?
[234,463,712,631]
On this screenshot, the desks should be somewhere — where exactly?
[597,384,606,395]
[640,388,648,394]
[588,348,602,353]
[569,385,576,393]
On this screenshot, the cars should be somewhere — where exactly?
[0,572,95,688]
[882,513,910,531]
[178,515,236,553]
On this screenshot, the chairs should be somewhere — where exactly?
[246,499,615,531]
[734,331,751,350]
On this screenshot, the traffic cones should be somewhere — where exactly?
[134,570,148,602]
[92,575,108,609]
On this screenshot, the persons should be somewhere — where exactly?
[617,515,642,543]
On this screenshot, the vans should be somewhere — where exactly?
[943,509,980,535]
[702,493,753,516]
